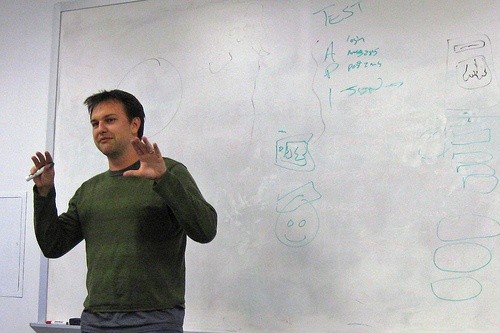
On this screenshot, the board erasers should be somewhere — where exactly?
[68,318,82,326]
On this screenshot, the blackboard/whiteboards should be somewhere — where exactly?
[27,0,500,333]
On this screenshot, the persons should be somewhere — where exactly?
[27,89,217,333]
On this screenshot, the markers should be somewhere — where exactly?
[25,162,55,182]
[44,320,69,325]
